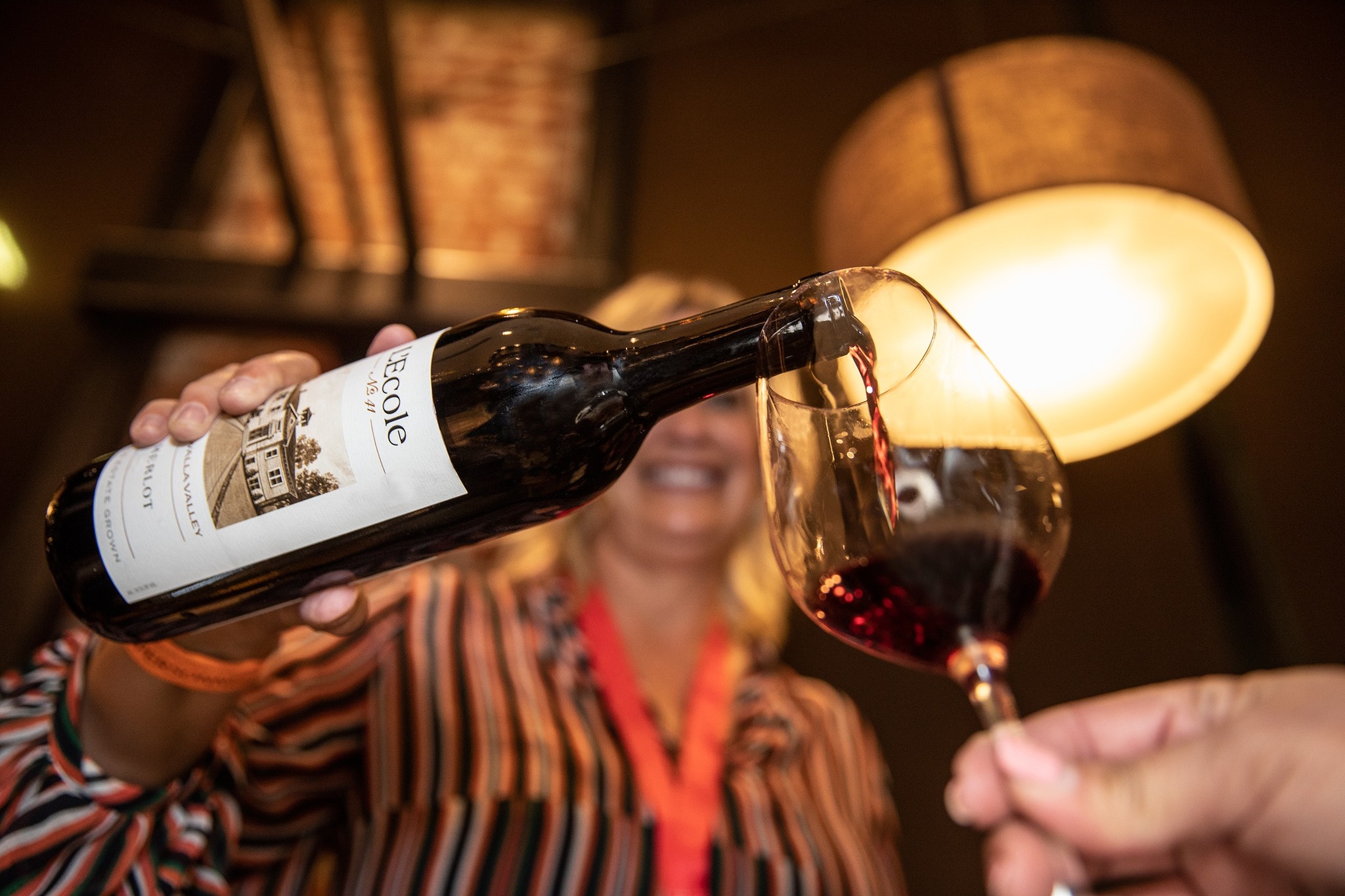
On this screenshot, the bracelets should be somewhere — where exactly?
[121,638,268,694]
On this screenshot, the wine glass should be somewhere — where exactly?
[759,265,1073,894]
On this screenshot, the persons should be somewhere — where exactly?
[944,664,1344,896]
[1,272,910,894]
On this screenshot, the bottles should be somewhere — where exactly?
[46,270,861,642]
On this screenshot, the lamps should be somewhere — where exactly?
[811,36,1277,465]
